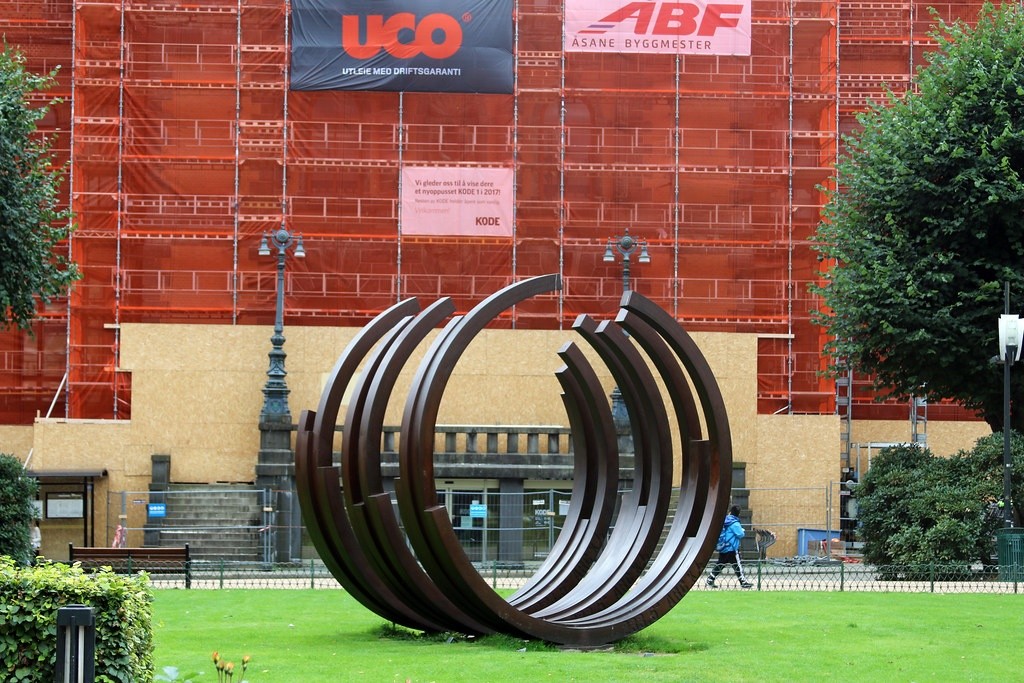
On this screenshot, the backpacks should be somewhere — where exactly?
[715,520,735,551]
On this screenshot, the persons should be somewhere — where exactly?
[31,521,41,565]
[705,505,754,588]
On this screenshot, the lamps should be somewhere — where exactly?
[55,604,96,683]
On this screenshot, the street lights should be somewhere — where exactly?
[600,227,652,427]
[257,227,307,423]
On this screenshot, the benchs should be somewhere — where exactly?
[68,542,193,589]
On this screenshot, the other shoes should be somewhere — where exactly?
[742,582,753,588]
[707,580,718,588]
